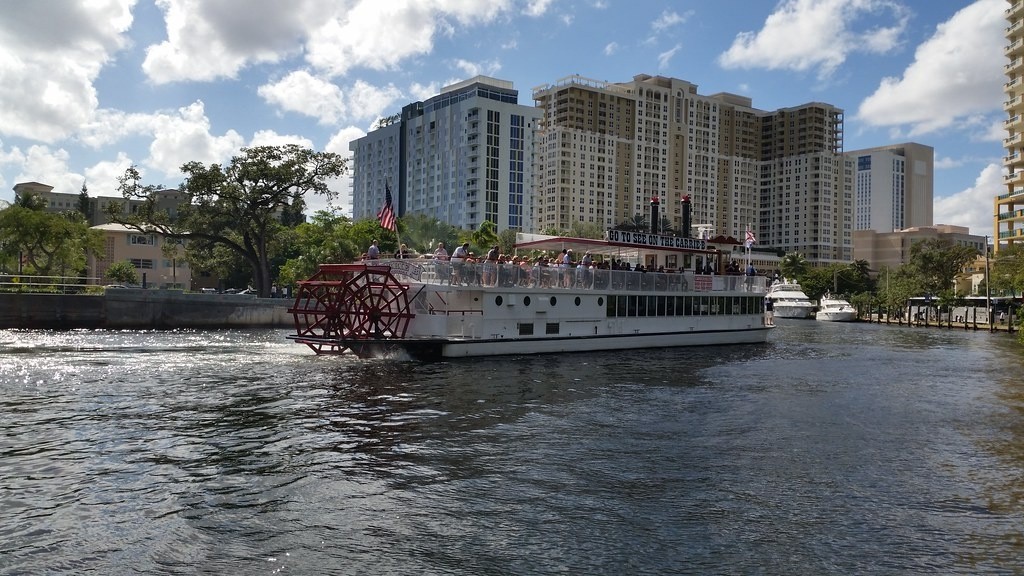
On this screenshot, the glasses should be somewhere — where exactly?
[402,246,405,247]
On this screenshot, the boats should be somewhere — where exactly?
[200,287,257,295]
[765,274,816,319]
[816,288,858,322]
[284,196,778,365]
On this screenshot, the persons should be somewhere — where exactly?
[360,238,758,291]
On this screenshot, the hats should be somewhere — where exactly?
[586,251,592,255]
[469,252,474,255]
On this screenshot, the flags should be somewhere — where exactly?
[376,182,398,232]
[745,231,756,243]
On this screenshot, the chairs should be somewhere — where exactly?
[368,261,687,291]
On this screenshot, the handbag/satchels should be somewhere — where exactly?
[751,266,755,276]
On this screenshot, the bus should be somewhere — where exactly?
[904,296,1024,325]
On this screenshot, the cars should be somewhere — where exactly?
[105,284,129,289]
